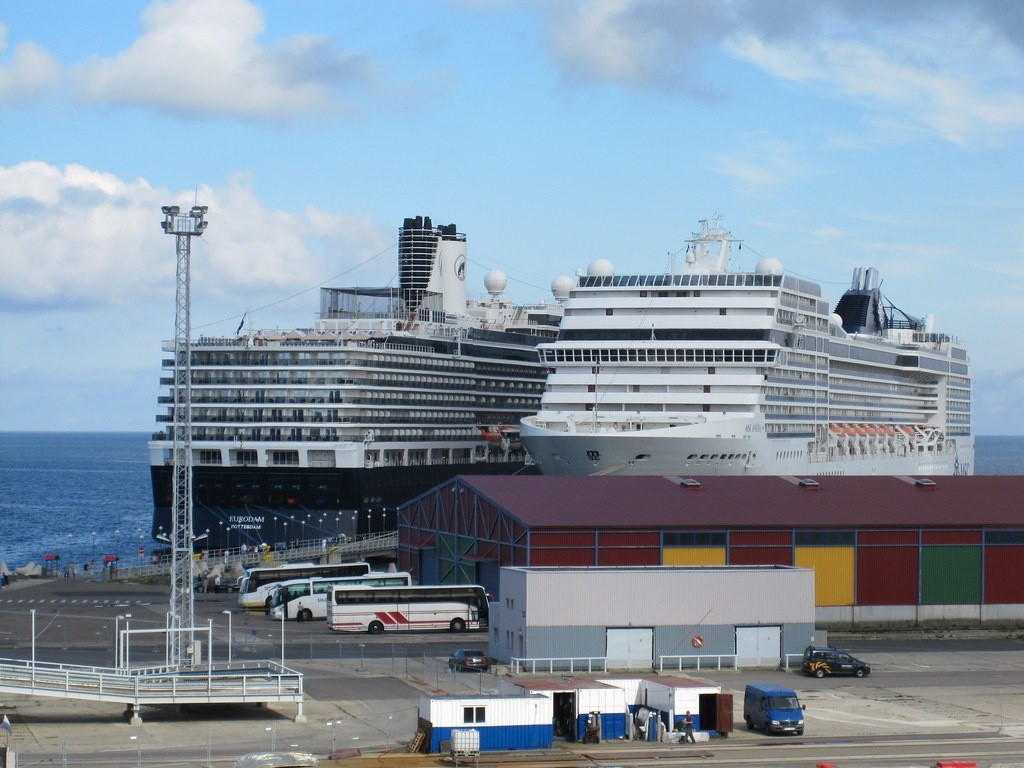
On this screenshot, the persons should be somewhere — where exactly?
[1,531,353,588]
[681,711,696,744]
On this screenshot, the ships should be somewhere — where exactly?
[519,214,976,489]
[136,187,578,550]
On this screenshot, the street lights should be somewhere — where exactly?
[67,534,75,577]
[113,529,121,579]
[91,531,99,571]
[137,501,411,576]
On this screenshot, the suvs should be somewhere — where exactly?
[803,645,871,678]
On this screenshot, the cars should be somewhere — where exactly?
[448,648,489,673]
[193,577,240,593]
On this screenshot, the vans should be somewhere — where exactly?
[743,683,806,737]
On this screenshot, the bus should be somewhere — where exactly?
[235,562,375,607]
[326,583,497,633]
[265,571,416,624]
